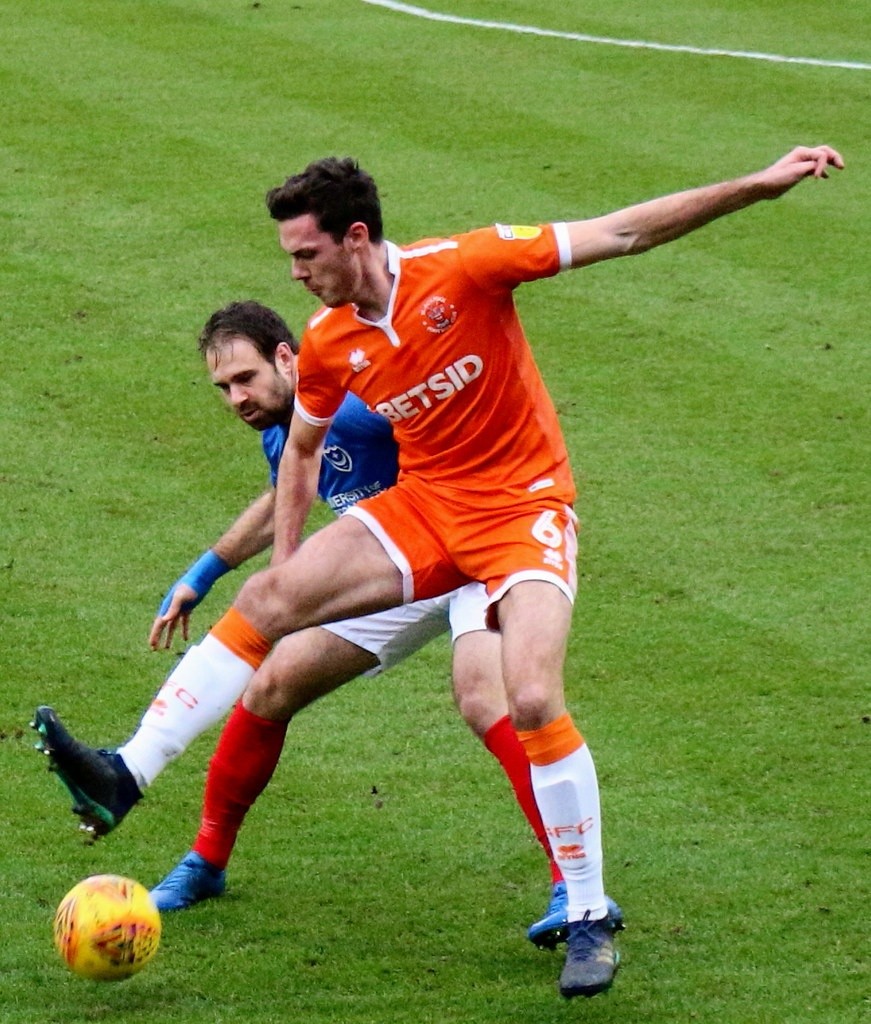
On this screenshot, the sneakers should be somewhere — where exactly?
[28,705,131,840]
[526,884,627,950]
[557,909,619,997]
[147,852,225,911]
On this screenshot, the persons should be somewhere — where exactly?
[30,144,846,1002]
[149,299,625,951]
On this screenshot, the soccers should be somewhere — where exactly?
[53,875,163,982]
